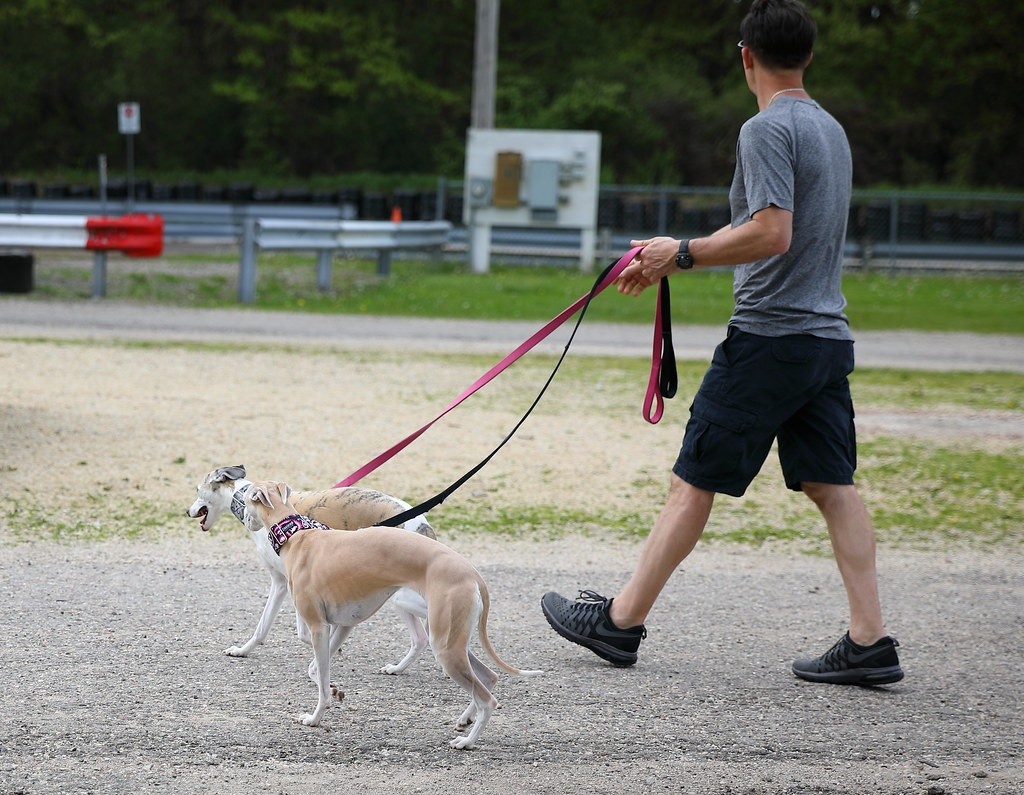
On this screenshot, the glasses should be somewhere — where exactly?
[738,41,746,49]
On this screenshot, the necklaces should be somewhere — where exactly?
[768,88,804,107]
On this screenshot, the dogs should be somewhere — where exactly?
[185,464,450,681]
[240,481,544,749]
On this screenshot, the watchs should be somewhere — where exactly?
[675,239,693,269]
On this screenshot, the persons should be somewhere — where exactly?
[541,0,905,685]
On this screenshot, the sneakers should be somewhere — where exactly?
[541,583,649,665]
[792,630,904,687]
